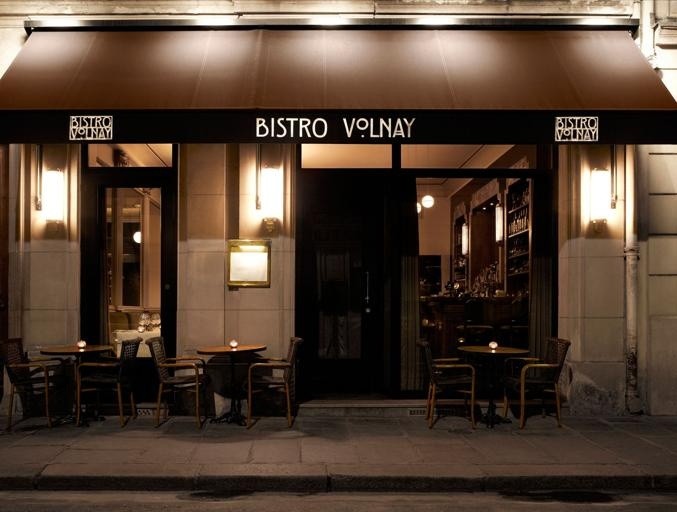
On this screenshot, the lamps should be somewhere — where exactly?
[42,145,72,238]
[253,143,288,235]
[588,168,612,240]
[422,195,434,209]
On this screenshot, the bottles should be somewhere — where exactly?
[510,207,528,236]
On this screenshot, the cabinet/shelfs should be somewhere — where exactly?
[447,141,534,303]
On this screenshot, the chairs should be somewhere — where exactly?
[2,336,68,428]
[75,336,143,429]
[418,337,571,431]
[144,337,212,430]
[247,337,303,429]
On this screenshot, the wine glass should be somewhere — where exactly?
[138,312,161,333]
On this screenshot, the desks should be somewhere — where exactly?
[115,327,162,360]
[40,343,115,428]
[196,343,268,427]
